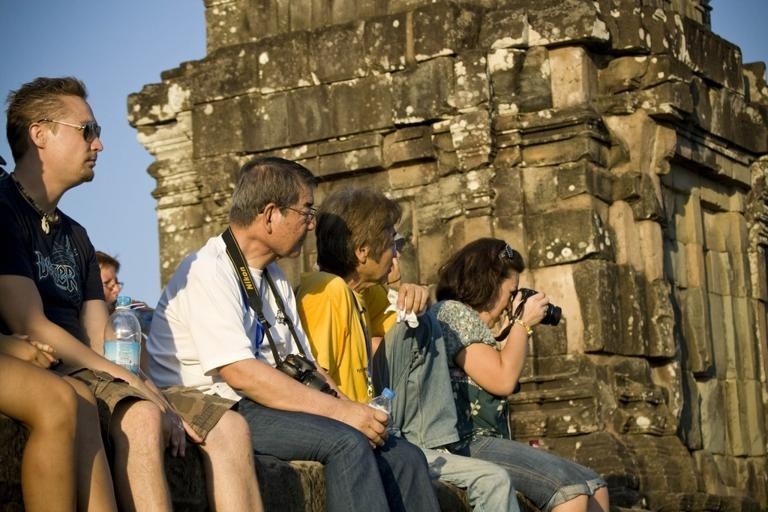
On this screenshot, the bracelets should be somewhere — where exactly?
[514,319,533,336]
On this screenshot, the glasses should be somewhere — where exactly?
[497,244,514,260]
[38,118,100,143]
[103,278,123,290]
[284,205,318,223]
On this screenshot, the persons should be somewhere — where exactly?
[298,189,520,512]
[0,77,265,512]
[428,238,610,512]
[0,333,118,512]
[95,251,154,343]
[145,157,442,512]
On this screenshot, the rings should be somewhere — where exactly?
[545,306,548,312]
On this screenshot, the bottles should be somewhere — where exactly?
[103,296,141,378]
[363,387,395,414]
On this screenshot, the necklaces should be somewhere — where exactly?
[10,172,59,235]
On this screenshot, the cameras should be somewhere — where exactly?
[275,354,338,397]
[512,288,562,327]
